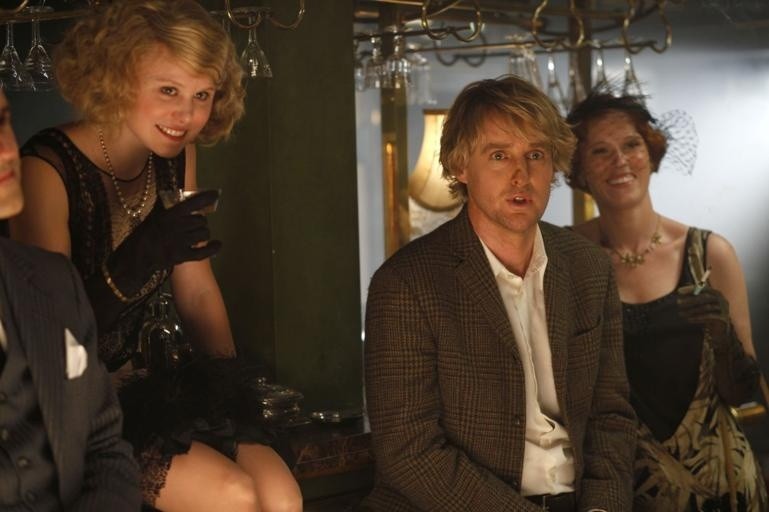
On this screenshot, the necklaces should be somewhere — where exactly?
[601,215,661,267]
[99,129,153,218]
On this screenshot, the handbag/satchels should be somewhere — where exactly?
[635,392,769,512]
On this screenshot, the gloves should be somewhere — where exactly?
[102,190,220,301]
[678,286,758,408]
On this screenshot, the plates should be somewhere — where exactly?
[241,377,363,424]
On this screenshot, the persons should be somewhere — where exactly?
[0,0,304,512]
[360,74,639,512]
[0,89,143,512]
[565,72,760,512]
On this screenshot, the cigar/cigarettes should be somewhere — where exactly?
[693,281,705,296]
[700,263,713,282]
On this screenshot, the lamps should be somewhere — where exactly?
[404,104,464,213]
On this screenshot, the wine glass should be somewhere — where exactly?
[388,26,411,92]
[1,13,34,97]
[239,8,277,81]
[509,37,649,119]
[25,16,62,93]
[361,32,391,89]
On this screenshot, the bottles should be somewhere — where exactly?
[138,295,190,367]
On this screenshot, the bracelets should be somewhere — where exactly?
[102,263,128,303]
